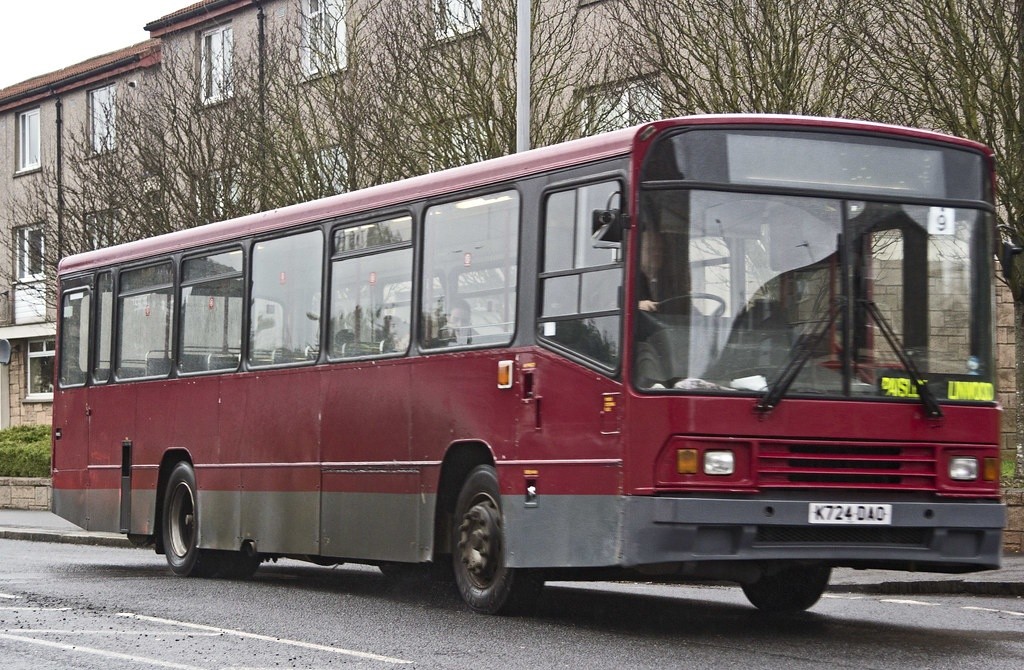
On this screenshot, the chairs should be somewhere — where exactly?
[94,321,515,382]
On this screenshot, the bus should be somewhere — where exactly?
[44,113,1008,616]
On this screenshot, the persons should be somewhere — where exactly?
[601,232,701,342]
[448,301,480,338]
